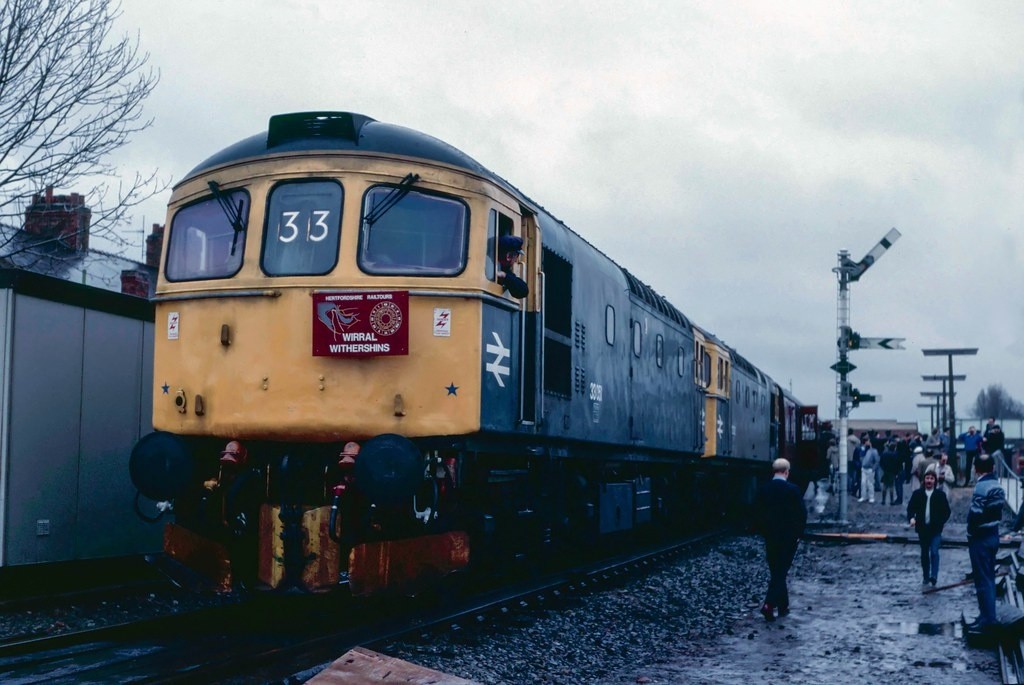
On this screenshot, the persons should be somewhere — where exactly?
[964,453,1003,630]
[847,423,904,505]
[982,416,1005,453]
[904,471,949,584]
[488,230,535,299]
[959,424,983,488]
[826,436,842,493]
[747,452,810,617]
[902,426,954,511]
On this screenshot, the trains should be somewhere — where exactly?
[132,110,835,629]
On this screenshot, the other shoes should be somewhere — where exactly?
[922,581,938,593]
[894,499,902,505]
[890,502,895,505]
[869,498,875,503]
[778,605,790,615]
[857,496,866,502]
[881,501,885,505]
[761,603,776,623]
[965,618,999,632]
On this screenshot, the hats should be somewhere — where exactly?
[914,447,923,453]
